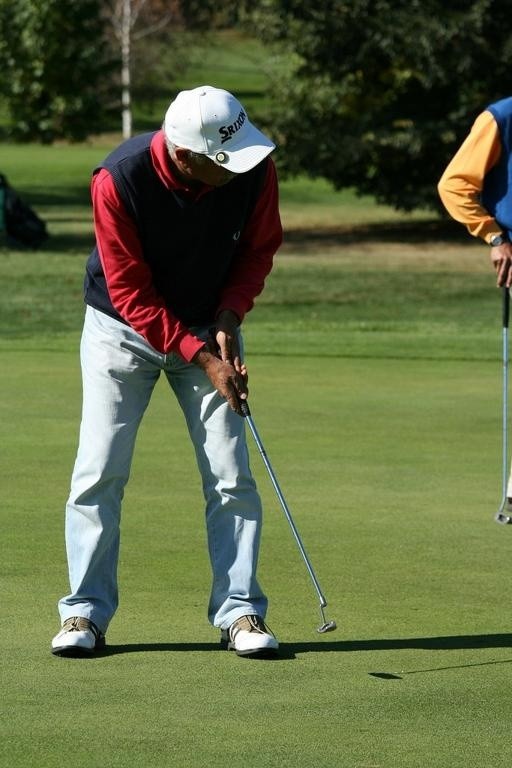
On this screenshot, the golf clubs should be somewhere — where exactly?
[495,276,512,524]
[237,387,336,634]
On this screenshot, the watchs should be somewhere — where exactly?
[487,234,507,247]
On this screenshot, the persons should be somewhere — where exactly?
[51,83,283,659]
[436,93,512,508]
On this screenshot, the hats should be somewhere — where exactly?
[165,86,277,174]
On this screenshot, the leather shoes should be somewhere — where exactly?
[52,616,105,654]
[221,615,279,655]
